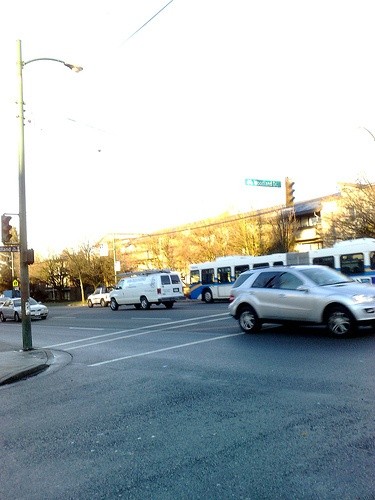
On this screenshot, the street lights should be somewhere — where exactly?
[16,40,84,353]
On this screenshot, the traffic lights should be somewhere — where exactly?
[1,215,13,243]
[285,177,296,207]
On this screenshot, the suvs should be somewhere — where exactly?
[229,264,375,338]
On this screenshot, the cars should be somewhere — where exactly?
[0,297,48,322]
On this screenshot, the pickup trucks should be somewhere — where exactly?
[86,287,114,308]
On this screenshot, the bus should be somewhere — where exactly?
[186,236,375,304]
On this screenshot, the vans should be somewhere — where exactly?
[104,272,184,310]
